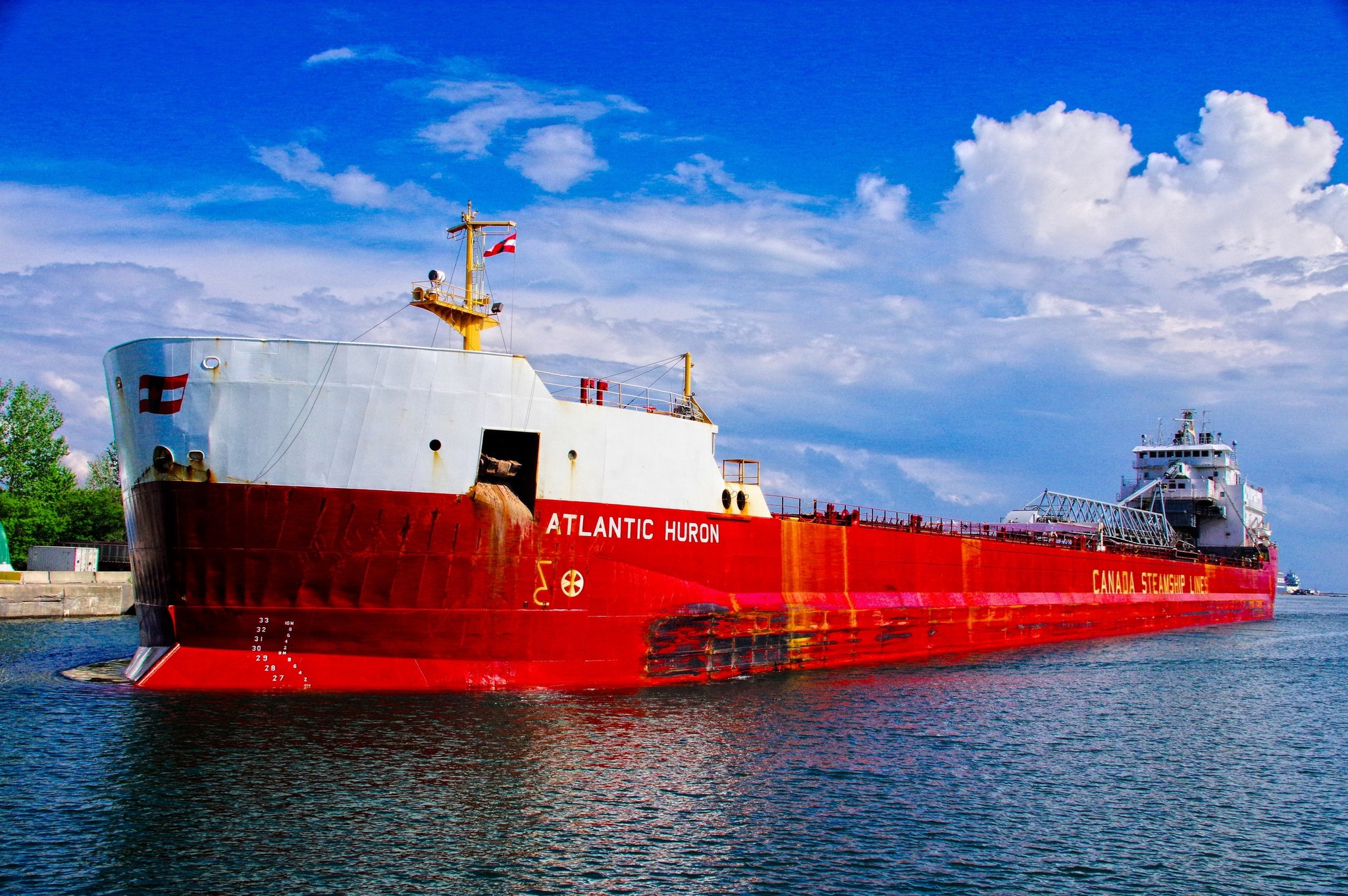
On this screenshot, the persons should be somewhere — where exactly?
[1250,555,1258,560]
[1245,555,1251,566]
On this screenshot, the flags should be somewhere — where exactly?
[483,232,516,257]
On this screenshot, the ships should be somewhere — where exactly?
[103,199,1277,705]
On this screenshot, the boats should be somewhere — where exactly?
[1278,569,1300,595]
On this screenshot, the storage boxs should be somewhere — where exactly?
[27,546,99,572]
